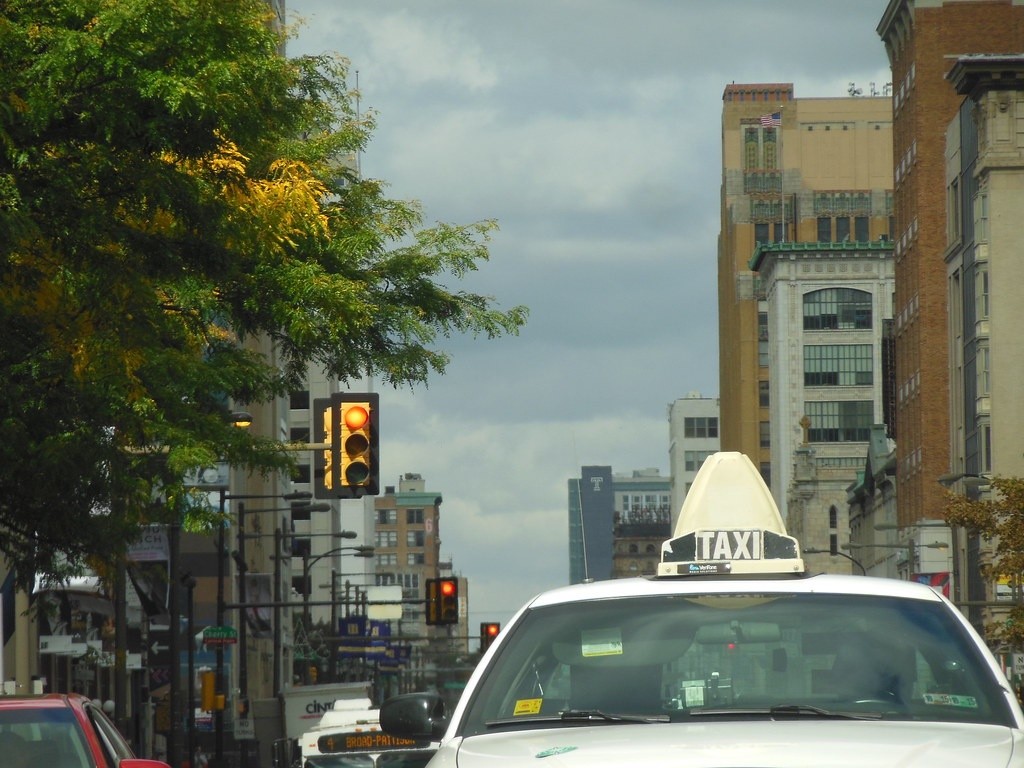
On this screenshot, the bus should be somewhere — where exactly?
[298,697,439,768]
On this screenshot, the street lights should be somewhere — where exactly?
[873,520,960,601]
[838,537,916,581]
[212,489,374,768]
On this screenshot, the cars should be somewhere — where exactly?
[380,451,1024,768]
[0,690,171,767]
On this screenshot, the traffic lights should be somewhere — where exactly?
[435,577,459,625]
[486,623,500,655]
[330,392,381,495]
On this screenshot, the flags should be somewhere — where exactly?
[761,111,783,126]
